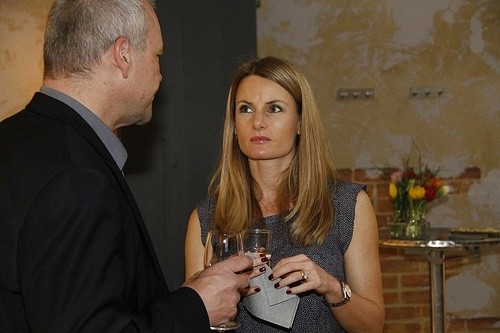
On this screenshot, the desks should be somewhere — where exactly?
[378,229,500,333]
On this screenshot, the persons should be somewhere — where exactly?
[0,0,253,333]
[185,56,385,333]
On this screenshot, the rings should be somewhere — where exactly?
[299,271,309,281]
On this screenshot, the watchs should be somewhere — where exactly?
[321,279,352,307]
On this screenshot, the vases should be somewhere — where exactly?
[385,220,431,240]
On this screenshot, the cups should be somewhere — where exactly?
[240,228,272,269]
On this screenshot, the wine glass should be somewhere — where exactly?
[203,229,244,330]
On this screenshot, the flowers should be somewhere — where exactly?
[378,135,451,219]
